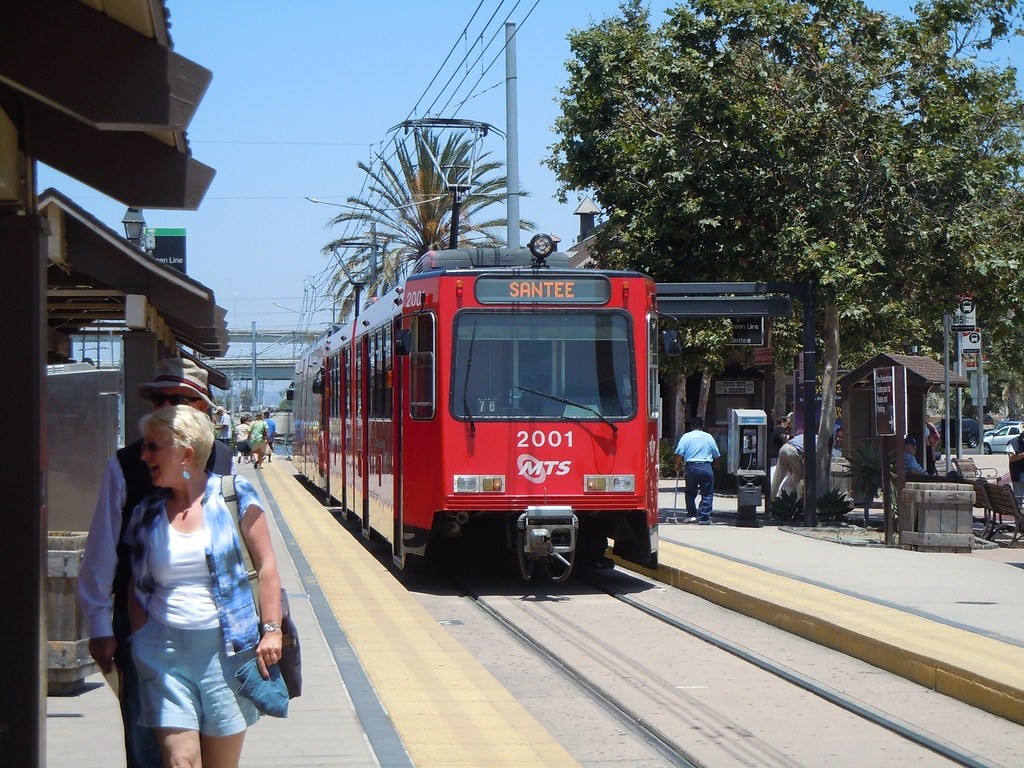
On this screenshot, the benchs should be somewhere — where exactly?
[950,456,1024,547]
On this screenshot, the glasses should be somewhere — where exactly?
[141,442,185,453]
[148,392,202,406]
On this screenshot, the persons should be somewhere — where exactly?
[925,421,940,476]
[48,351,77,364]
[1006,422,1024,508]
[123,404,286,768]
[216,406,276,469]
[81,358,94,366]
[831,429,843,460]
[74,359,239,768]
[904,434,930,475]
[675,417,721,525]
[771,401,818,515]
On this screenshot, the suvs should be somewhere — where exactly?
[933,418,979,448]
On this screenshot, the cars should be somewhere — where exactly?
[983,420,1024,455]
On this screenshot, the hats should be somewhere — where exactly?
[904,437,918,445]
[214,406,224,413]
[235,657,289,718]
[136,357,218,410]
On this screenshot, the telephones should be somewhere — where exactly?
[744,429,757,453]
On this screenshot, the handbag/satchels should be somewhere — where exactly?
[264,444,272,455]
[262,426,266,436]
[221,474,303,698]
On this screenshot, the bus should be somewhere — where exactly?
[287,235,661,584]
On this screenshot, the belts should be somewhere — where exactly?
[686,462,710,465]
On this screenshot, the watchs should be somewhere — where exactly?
[261,621,281,632]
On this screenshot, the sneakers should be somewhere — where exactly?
[683,516,696,523]
[699,520,712,525]
